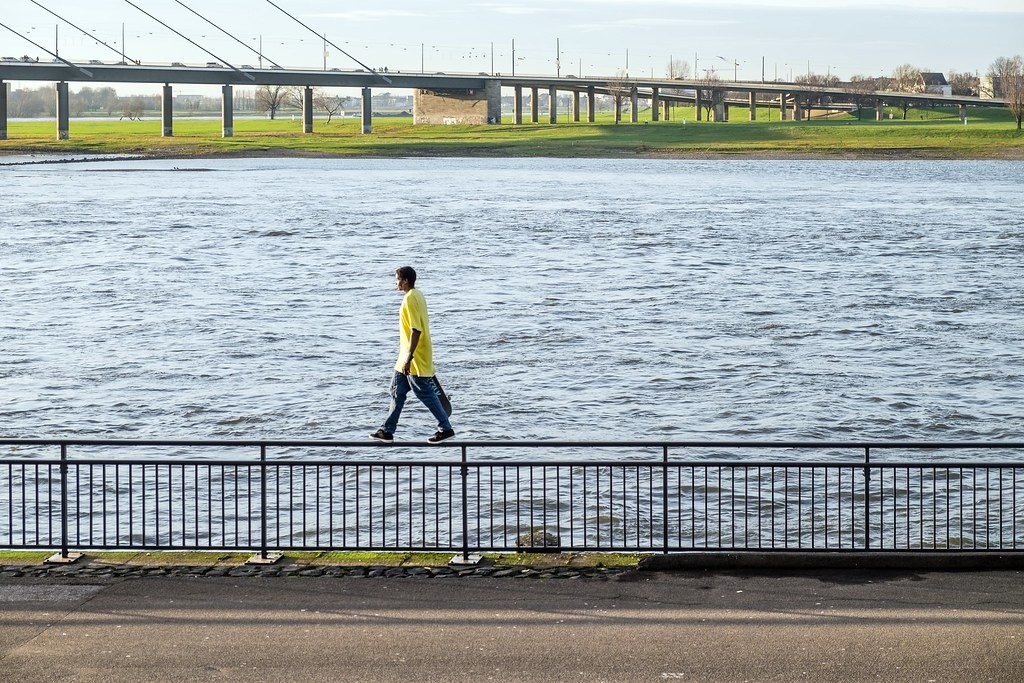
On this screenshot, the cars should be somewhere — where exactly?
[241,65,255,69]
[435,71,447,76]
[116,61,128,64]
[86,59,103,63]
[354,68,365,72]
[566,74,579,79]
[331,67,341,72]
[1,55,36,63]
[170,62,186,67]
[477,72,490,76]
[269,65,284,70]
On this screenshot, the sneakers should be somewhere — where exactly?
[428,427,456,444]
[369,429,393,443]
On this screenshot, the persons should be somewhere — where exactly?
[369,266,455,444]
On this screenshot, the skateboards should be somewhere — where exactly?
[423,374,453,419]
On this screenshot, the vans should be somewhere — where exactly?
[205,62,224,68]
